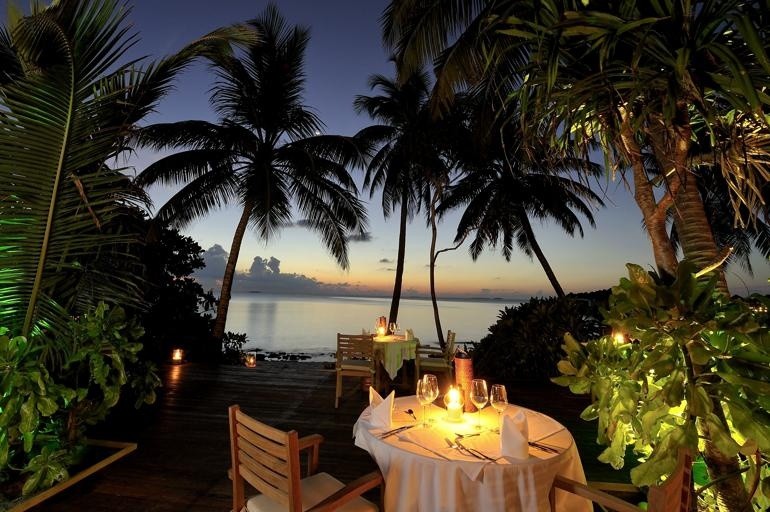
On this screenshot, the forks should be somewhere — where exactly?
[444,437,496,463]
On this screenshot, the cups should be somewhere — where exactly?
[362,327,371,336]
[172,348,183,364]
[247,352,257,367]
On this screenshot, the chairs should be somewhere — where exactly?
[548,444,692,512]
[334,329,456,409]
[228,404,382,512]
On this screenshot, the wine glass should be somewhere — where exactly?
[390,323,401,340]
[470,379,509,433]
[416,373,438,431]
